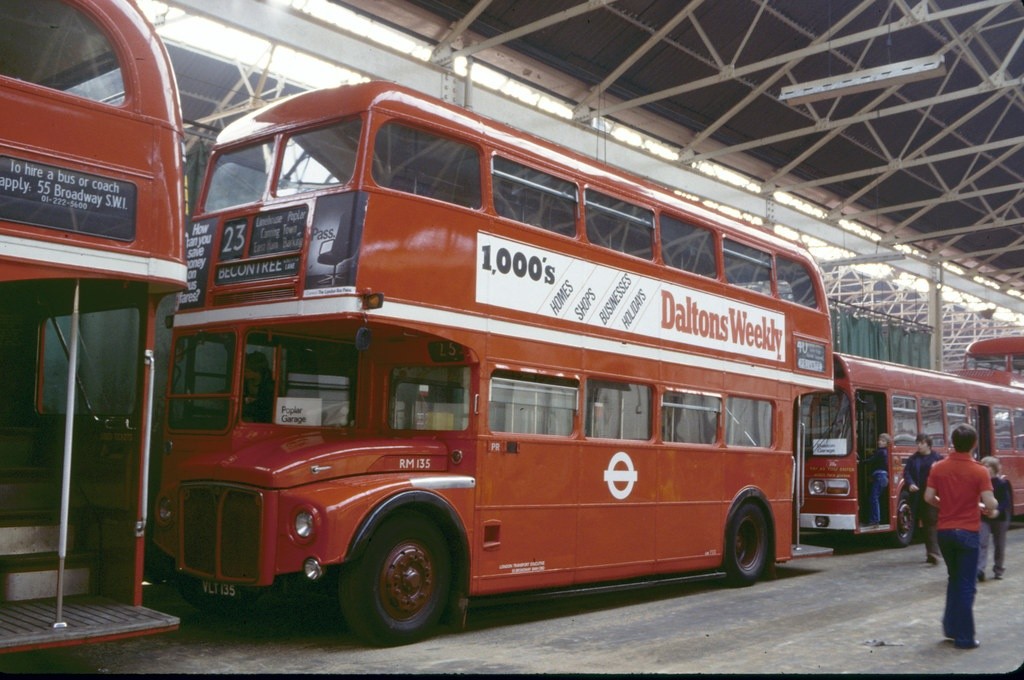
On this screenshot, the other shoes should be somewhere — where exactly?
[946,634,980,648]
[995,574,1003,580]
[977,570,984,582]
[927,553,939,566]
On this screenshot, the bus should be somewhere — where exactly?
[795,336,1024,547]
[157,80,836,649]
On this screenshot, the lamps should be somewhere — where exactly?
[777,54,947,104]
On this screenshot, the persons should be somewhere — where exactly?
[855,433,891,529]
[243,350,275,420]
[903,433,945,566]
[976,457,1012,582]
[924,424,999,650]
